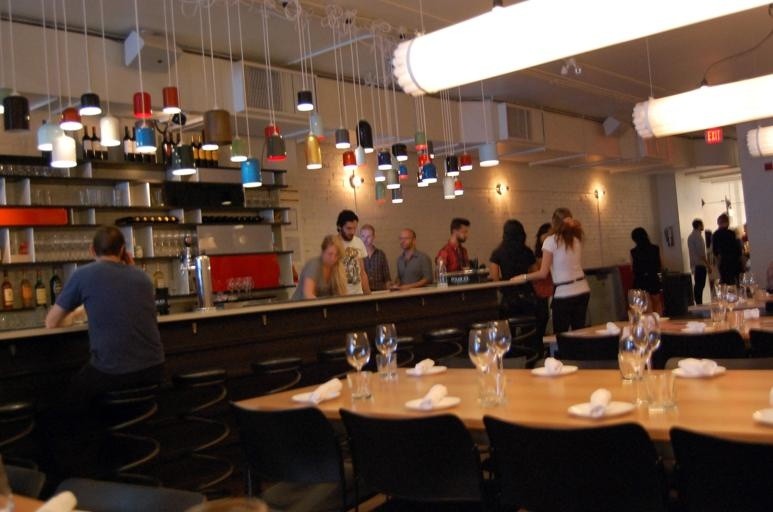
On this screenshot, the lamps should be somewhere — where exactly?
[392,0,773,99]
[295,2,500,205]
[745,124,772,159]
[1,1,31,134]
[633,39,772,138]
[36,1,288,188]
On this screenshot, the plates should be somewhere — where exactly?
[752,408,773,426]
[530,365,578,377]
[405,365,448,377]
[659,317,670,323]
[671,366,726,377]
[404,396,461,411]
[291,391,341,404]
[567,401,634,419]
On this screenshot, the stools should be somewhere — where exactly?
[500,315,538,370]
[177,366,233,499]
[319,341,367,463]
[96,385,163,488]
[252,353,302,471]
[0,403,40,500]
[423,326,463,368]
[395,333,415,368]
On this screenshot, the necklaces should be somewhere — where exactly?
[524,273,528,282]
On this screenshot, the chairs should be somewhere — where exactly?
[481,414,668,511]
[667,424,772,509]
[552,329,624,367]
[653,326,748,369]
[230,401,351,509]
[748,330,771,359]
[5,465,50,497]
[338,405,492,509]
[54,477,208,511]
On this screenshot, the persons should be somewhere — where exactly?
[291,236,344,302]
[359,225,391,291]
[528,223,556,356]
[488,219,537,339]
[629,228,663,320]
[507,207,591,357]
[688,214,750,306]
[436,218,469,284]
[381,228,432,291]
[45,225,165,421]
[335,210,372,297]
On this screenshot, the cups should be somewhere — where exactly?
[0,164,122,205]
[468,369,509,405]
[618,352,678,413]
[708,271,758,332]
[347,353,398,401]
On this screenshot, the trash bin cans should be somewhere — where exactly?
[662,272,690,316]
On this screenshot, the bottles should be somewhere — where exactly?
[436,255,448,288]
[41,120,218,168]
[115,215,176,227]
[141,261,166,289]
[1,266,64,311]
[202,216,262,226]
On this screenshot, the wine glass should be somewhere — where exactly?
[346,323,398,395]
[34,231,95,262]
[468,320,512,405]
[227,277,254,297]
[628,288,661,399]
[152,228,198,256]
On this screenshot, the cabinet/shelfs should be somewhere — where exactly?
[0,154,297,331]
